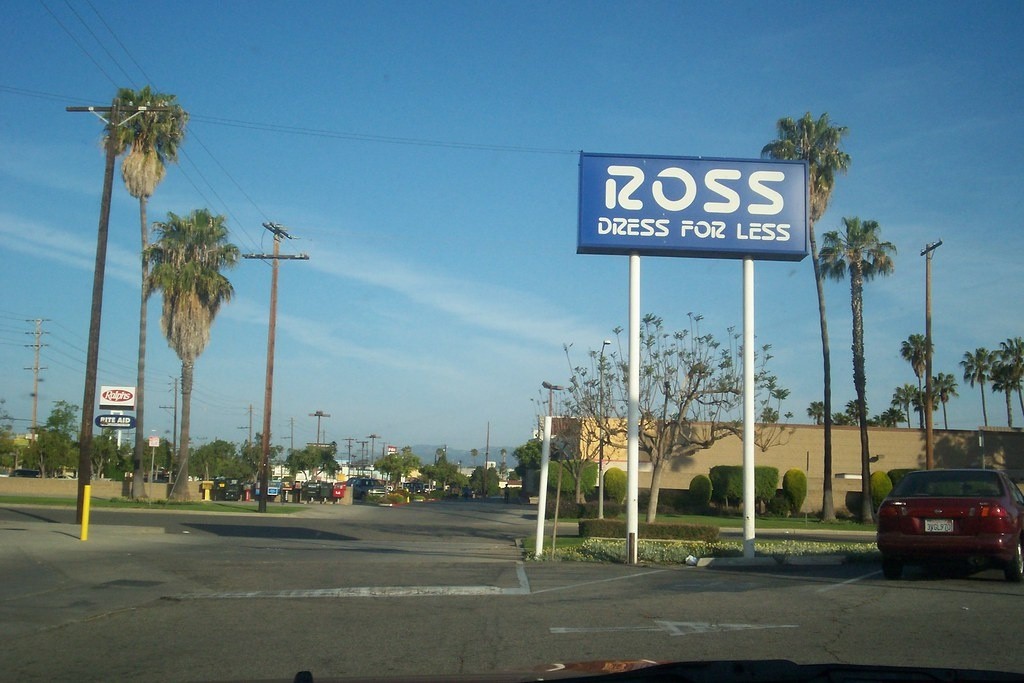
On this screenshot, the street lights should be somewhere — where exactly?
[309,410,331,476]
[356,440,369,464]
[366,434,381,465]
[542,381,564,415]
[599,341,611,518]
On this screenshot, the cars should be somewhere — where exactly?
[346,478,385,500]
[877,469,1024,582]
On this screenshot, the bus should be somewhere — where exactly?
[403,483,444,496]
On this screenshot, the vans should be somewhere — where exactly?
[9,469,40,478]
[222,477,241,500]
